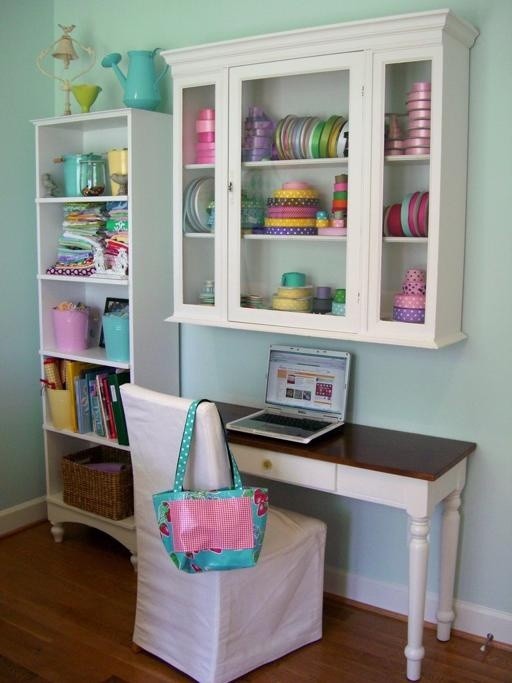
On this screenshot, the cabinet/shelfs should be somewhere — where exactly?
[32,106,185,574]
[223,14,365,344]
[365,9,479,351]
[161,39,222,331]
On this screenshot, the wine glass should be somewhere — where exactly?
[69,84,102,112]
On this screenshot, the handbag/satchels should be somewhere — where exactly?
[152,487,269,574]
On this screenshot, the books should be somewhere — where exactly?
[65,361,130,445]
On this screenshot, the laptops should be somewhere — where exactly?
[226,344,351,445]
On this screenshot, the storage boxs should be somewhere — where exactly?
[114,382,328,681]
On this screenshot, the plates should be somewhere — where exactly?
[184,174,215,233]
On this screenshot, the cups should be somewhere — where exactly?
[46,385,73,431]
[53,305,91,354]
[101,309,129,363]
[108,147,128,195]
[63,151,103,196]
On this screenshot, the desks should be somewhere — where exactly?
[193,398,478,682]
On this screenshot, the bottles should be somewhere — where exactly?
[78,160,106,196]
[197,280,214,304]
